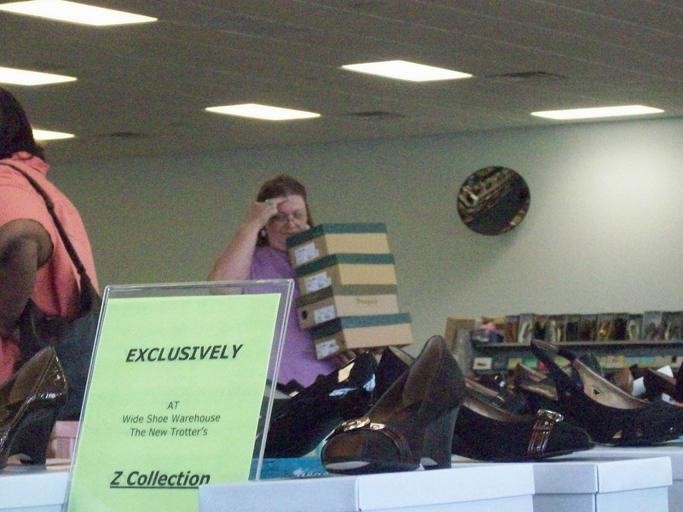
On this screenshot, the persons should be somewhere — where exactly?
[0,87,103,422]
[207,176,383,388]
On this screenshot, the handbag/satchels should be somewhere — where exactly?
[0,161,103,421]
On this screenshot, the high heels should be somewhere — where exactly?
[643,366,683,404]
[264,351,379,458]
[320,335,466,475]
[675,361,683,394]
[629,364,640,379]
[514,362,548,386]
[463,365,517,414]
[538,350,601,385]
[286,379,305,390]
[608,367,634,396]
[530,338,681,447]
[518,380,559,415]
[266,379,300,399]
[375,345,595,462]
[632,365,673,402]
[0,346,68,469]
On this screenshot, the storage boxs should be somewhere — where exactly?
[310,312,414,361]
[534,456,675,512]
[199,461,537,512]
[294,283,400,329]
[294,253,398,296]
[287,222,392,270]
[0,458,71,512]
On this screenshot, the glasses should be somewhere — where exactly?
[273,211,307,221]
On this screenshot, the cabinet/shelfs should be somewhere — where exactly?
[444,311,683,374]
